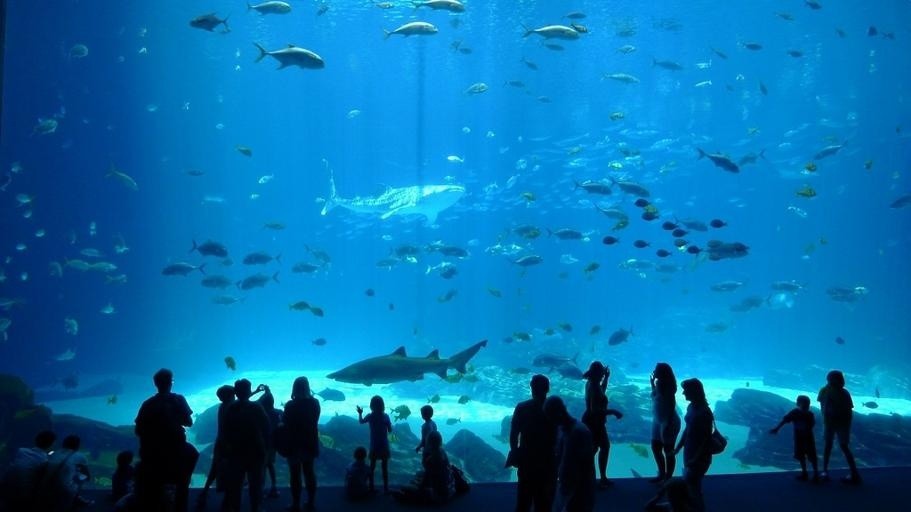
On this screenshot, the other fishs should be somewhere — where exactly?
[0,0,910,482]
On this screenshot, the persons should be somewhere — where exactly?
[2,365,453,512]
[816,369,864,488]
[507,360,624,512]
[767,394,819,482]
[642,476,703,512]
[668,377,716,512]
[648,363,681,484]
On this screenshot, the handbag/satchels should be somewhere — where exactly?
[710,430,727,454]
